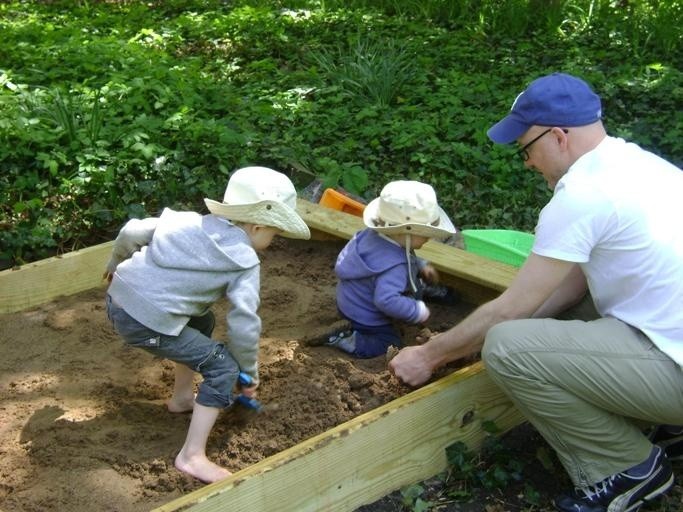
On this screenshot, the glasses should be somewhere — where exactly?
[516,128,568,161]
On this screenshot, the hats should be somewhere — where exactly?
[204,167,311,241]
[363,181,456,238]
[487,72,601,143]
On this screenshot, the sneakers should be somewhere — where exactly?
[305,320,353,347]
[418,278,452,301]
[552,445,674,512]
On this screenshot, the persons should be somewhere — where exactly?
[321,179,462,360]
[386,71,683,511]
[100,166,311,485]
